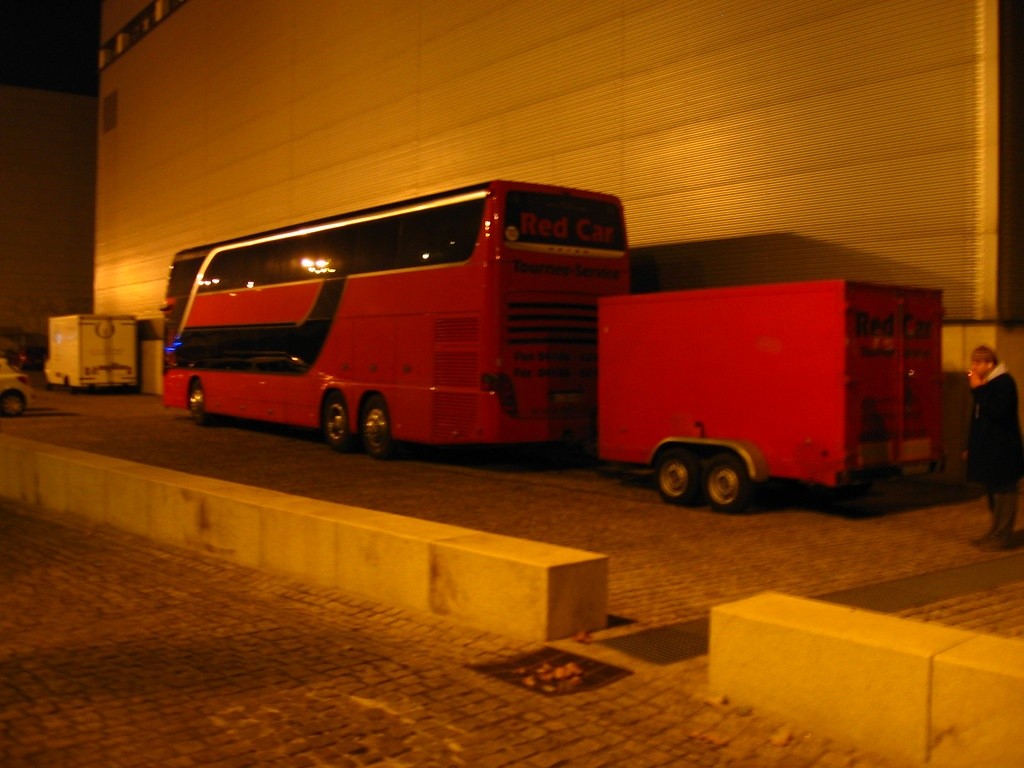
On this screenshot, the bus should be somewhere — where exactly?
[157,180,629,461]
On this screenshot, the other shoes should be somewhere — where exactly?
[981,539,1013,552]
[971,533,995,546]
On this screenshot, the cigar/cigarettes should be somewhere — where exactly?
[968,374,972,378]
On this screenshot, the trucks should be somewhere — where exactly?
[41,312,137,391]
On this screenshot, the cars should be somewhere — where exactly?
[0,359,37,418]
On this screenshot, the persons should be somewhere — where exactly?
[960,346,1024,550]
